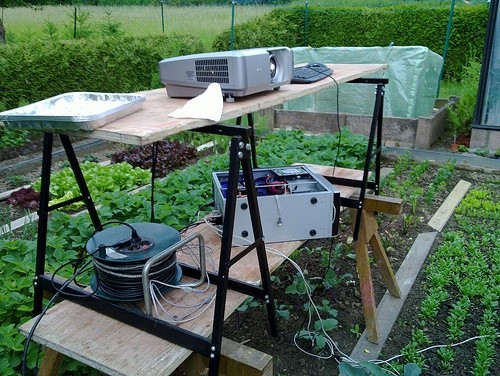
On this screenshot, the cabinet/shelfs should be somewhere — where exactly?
[19,62,403,376]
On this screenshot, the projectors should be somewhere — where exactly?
[157,46,294,103]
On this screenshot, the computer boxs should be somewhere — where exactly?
[210,163,341,246]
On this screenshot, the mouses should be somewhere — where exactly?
[306,63,324,67]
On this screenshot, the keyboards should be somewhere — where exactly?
[290,66,333,84]
